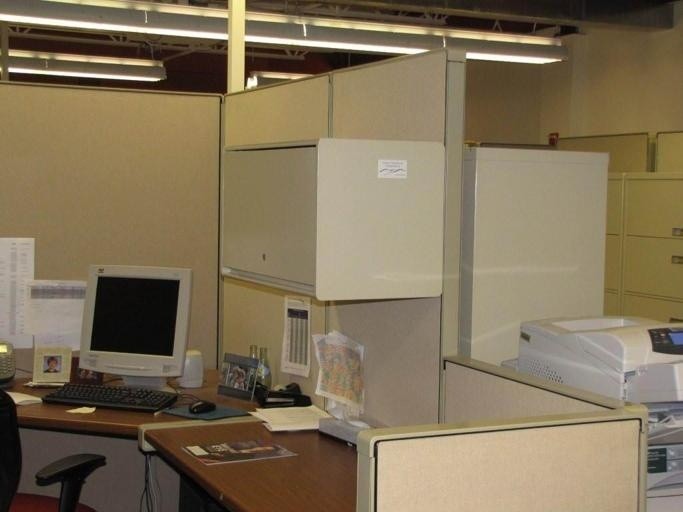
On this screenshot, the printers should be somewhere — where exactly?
[517,313,683,497]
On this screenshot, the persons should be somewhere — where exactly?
[233,369,246,390]
[197,443,282,464]
[227,367,239,388]
[43,356,60,373]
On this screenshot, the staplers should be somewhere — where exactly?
[263,391,311,407]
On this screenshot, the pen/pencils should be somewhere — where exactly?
[153,405,174,416]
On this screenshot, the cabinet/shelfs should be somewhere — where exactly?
[606,171,683,323]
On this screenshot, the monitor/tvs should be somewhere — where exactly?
[77,263,194,393]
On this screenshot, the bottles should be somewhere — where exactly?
[248,343,272,391]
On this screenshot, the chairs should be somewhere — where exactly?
[0,388,106,512]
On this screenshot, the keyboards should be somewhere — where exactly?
[41,380,179,415]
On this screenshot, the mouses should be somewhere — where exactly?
[189,399,216,415]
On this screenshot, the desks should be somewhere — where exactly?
[138,417,358,511]
[6,370,261,440]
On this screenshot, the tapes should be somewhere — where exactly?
[273,384,287,392]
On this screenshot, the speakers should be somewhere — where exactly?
[177,348,204,389]
